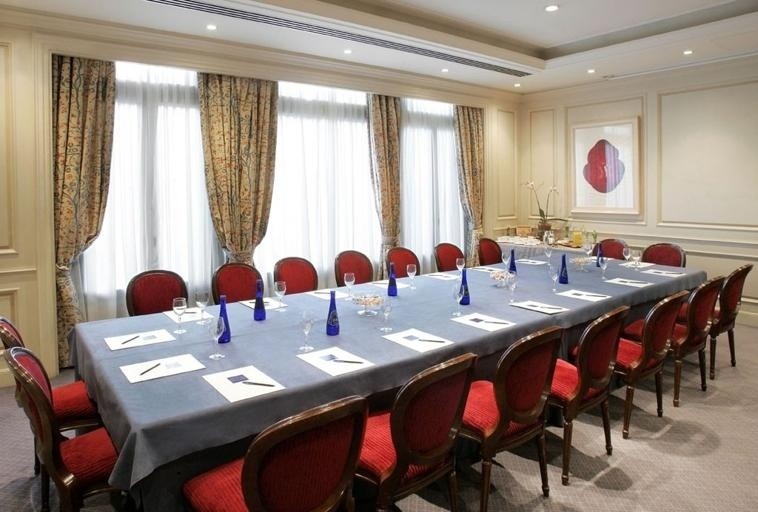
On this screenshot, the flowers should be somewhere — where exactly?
[525,176,562,223]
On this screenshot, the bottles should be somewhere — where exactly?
[558,252,571,285]
[508,248,521,275]
[326,289,340,337]
[596,243,603,267]
[458,268,470,306]
[216,294,232,344]
[387,263,399,297]
[252,279,267,322]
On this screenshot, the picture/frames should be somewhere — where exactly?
[570,117,643,219]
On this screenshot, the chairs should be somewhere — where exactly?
[592,239,633,261]
[573,289,692,441]
[478,238,503,268]
[457,323,565,512]
[271,255,319,297]
[186,394,366,511]
[552,305,632,484]
[124,269,188,317]
[354,351,478,512]
[1,319,99,476]
[386,246,420,280]
[679,261,753,379]
[643,242,688,268]
[627,275,724,405]
[212,261,263,306]
[3,347,128,510]
[432,242,465,273]
[335,250,372,287]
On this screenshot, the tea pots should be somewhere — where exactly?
[542,230,556,245]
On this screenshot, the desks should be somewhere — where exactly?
[74,253,707,511]
[497,233,594,259]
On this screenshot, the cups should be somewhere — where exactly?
[572,227,583,245]
[497,233,536,245]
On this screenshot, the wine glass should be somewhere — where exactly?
[456,257,465,271]
[548,266,561,293]
[376,296,394,333]
[405,264,417,290]
[205,317,227,361]
[273,280,288,313]
[504,273,520,304]
[342,272,357,302]
[582,241,594,259]
[297,308,317,354]
[542,246,555,267]
[501,251,511,268]
[193,289,210,326]
[598,256,609,280]
[451,283,465,317]
[172,297,188,335]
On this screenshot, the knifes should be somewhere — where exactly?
[484,321,508,326]
[333,359,363,365]
[518,259,529,263]
[419,338,445,343]
[430,273,444,277]
[247,298,270,304]
[473,265,485,271]
[312,290,331,295]
[586,292,606,298]
[186,310,197,316]
[139,363,161,377]
[373,282,389,285]
[242,381,275,389]
[539,305,562,311]
[622,246,643,267]
[121,334,140,345]
[628,281,648,285]
[666,271,683,275]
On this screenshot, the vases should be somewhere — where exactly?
[538,223,552,237]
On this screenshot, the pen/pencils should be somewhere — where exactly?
[140,363,161,375]
[243,381,274,387]
[587,295,606,297]
[430,274,443,276]
[185,311,196,313]
[419,340,444,343]
[592,259,597,260]
[484,321,507,324]
[629,281,648,284]
[518,261,529,262]
[250,302,268,304]
[666,272,683,274]
[540,307,562,309]
[472,268,485,270]
[373,283,386,285]
[334,360,364,364]
[121,335,141,344]
[315,292,329,294]
